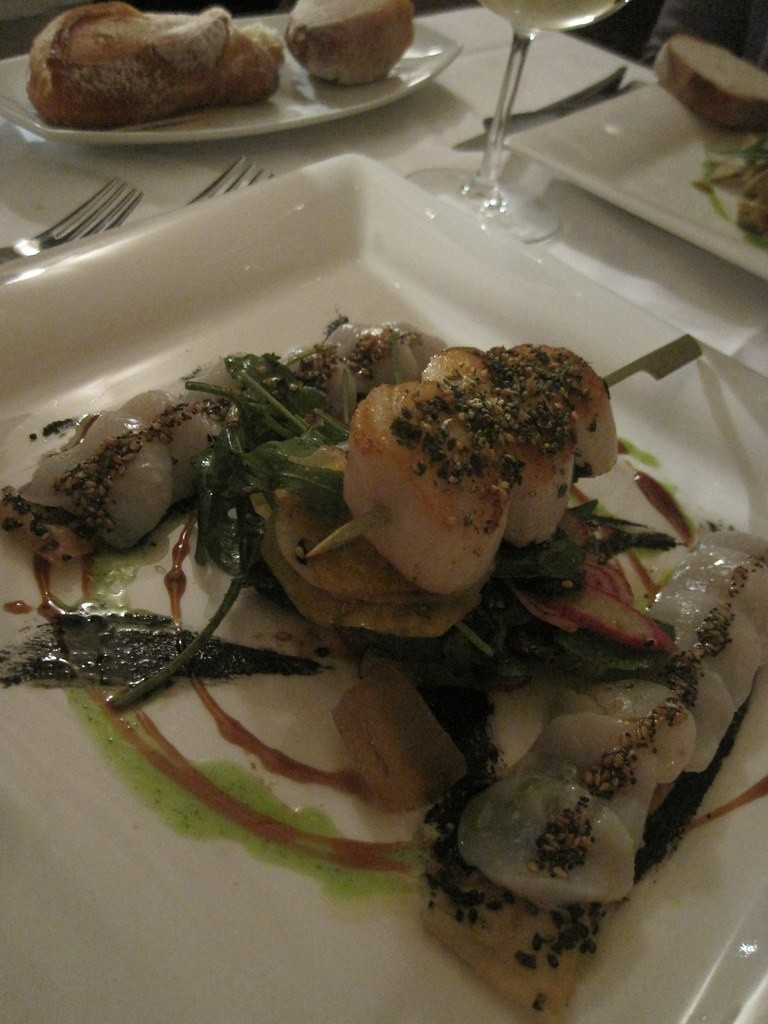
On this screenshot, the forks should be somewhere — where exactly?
[188,155,274,204]
[0,175,143,264]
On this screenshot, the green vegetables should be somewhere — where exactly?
[110,344,679,708]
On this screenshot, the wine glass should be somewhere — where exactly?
[404,1,628,244]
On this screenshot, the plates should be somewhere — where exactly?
[0,155,768,1024]
[503,83,768,284]
[0,14,462,147]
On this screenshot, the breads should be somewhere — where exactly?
[23,2,280,127]
[284,0,414,87]
[654,33,768,130]
